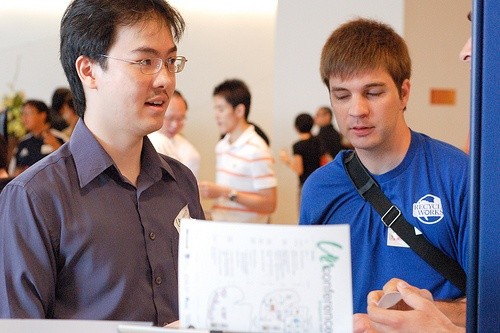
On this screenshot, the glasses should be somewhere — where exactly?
[90,53,188,75]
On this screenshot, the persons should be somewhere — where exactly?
[0,0,207,326]
[314,108,343,158]
[147,90,201,181]
[366,6,473,333]
[5,86,84,180]
[299,18,472,333]
[279,114,331,184]
[199,78,278,223]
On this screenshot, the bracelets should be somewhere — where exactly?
[230,190,237,201]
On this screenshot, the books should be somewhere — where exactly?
[177,217,353,333]
